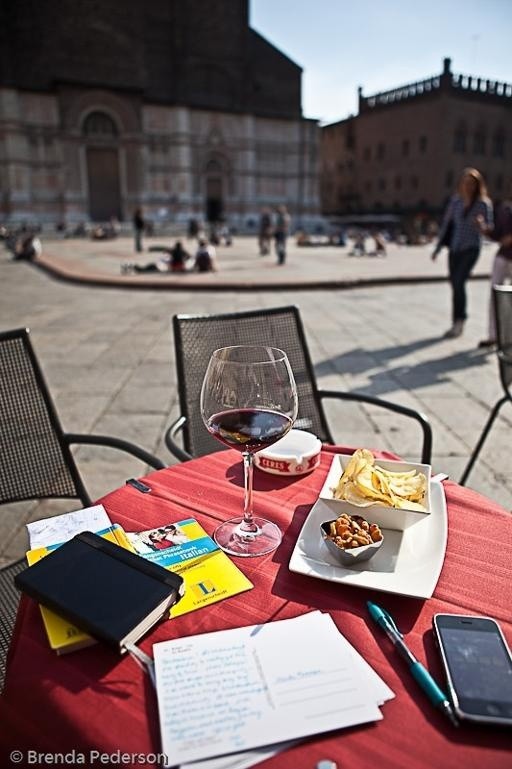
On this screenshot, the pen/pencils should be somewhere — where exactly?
[366,600,461,729]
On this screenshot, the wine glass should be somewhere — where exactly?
[199,344,300,557]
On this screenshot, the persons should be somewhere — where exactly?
[477,198,512,346]
[1,205,439,273]
[431,168,496,339]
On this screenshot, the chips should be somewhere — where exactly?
[334,446,425,508]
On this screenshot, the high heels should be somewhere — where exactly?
[444,327,462,337]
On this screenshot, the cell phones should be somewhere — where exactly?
[432,613,512,725]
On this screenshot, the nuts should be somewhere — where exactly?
[326,513,382,550]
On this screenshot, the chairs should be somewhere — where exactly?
[0,327,165,695]
[457,284,512,486]
[165,304,431,466]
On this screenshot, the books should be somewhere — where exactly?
[24,517,255,658]
[13,531,183,658]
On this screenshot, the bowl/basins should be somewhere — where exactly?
[319,519,385,567]
[319,454,431,530]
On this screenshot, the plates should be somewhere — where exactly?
[287,451,454,600]
